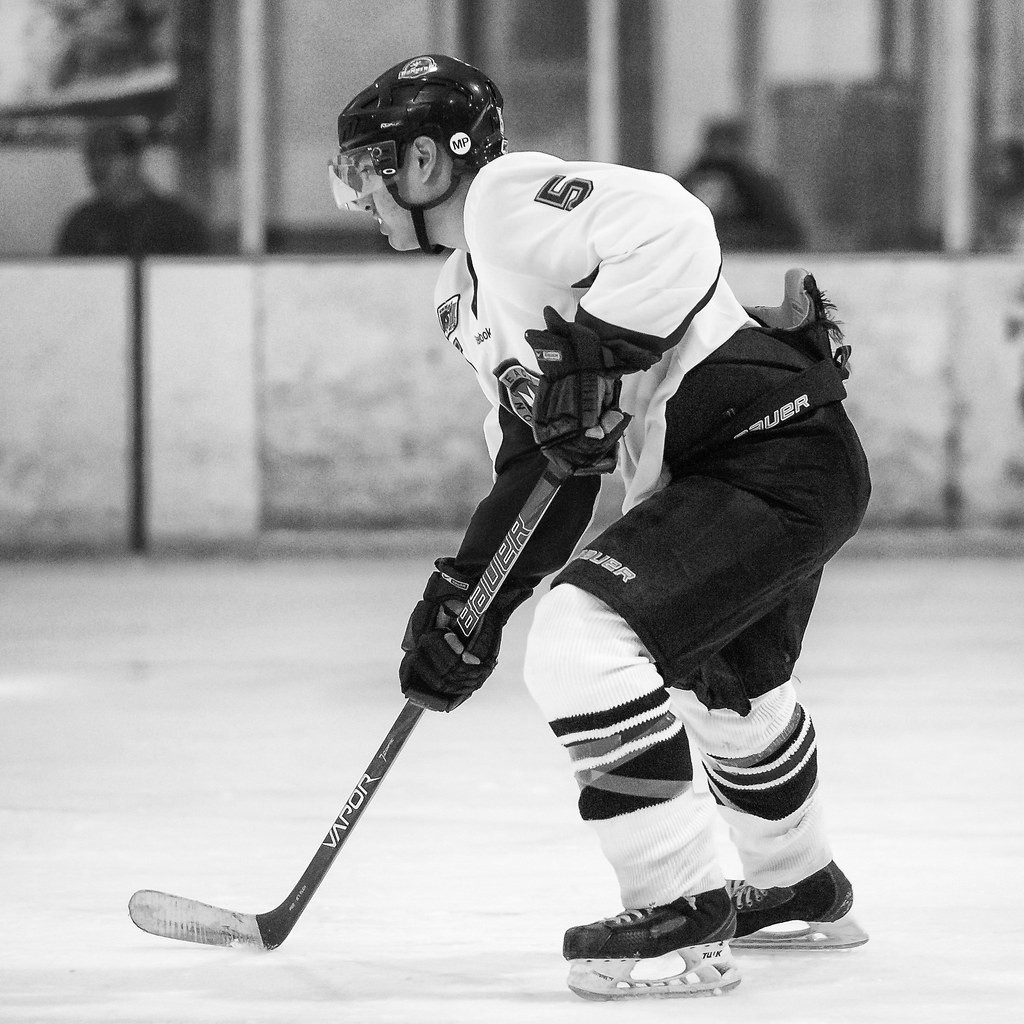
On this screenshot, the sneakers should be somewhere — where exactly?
[562,886,741,1002]
[727,860,869,950]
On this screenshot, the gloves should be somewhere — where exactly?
[400,557,533,713]
[525,306,663,475]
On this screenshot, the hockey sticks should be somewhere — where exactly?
[127,459,583,950]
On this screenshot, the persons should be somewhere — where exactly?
[675,118,812,255]
[972,135,1023,252]
[337,51,873,963]
[49,121,220,258]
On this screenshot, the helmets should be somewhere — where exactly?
[334,55,505,193]
